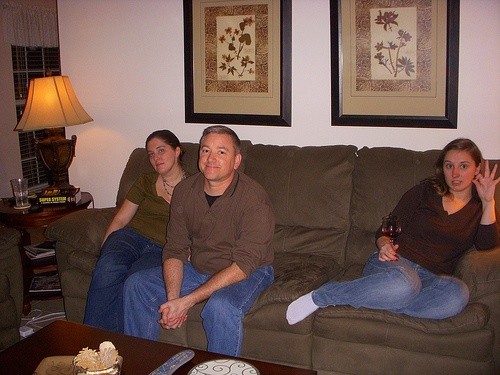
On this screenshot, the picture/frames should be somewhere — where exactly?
[183,0,292,127]
[330,0,460,129]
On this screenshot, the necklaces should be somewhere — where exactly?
[162,174,184,196]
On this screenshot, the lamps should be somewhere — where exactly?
[12,67,94,191]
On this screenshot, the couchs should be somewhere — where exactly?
[43,139,500,375]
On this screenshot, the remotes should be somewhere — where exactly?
[149,349,195,375]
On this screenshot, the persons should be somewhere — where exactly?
[83,129,192,341]
[122,125,276,357]
[285,138,500,325]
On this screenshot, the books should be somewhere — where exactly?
[24,240,56,260]
[37,188,81,204]
[29,276,61,292]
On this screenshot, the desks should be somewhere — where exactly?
[0,320,317,375]
[0,192,93,316]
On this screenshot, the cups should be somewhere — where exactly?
[9,177,29,207]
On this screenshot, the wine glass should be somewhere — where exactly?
[382,215,402,245]
